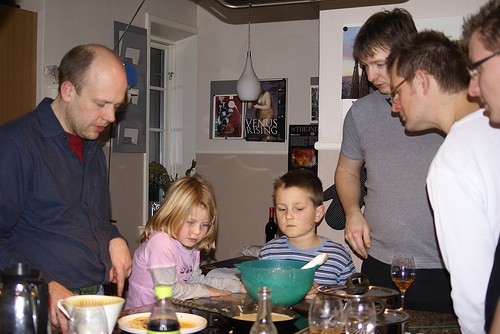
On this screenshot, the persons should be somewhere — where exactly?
[0,44,132,334]
[387,30,500,334]
[248,91,274,128]
[335,8,454,314]
[258,170,356,300]
[217,100,241,137]
[460,0,500,334]
[124,175,232,311]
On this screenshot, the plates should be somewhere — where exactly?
[117,312,207,334]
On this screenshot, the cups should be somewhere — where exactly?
[343,298,377,334]
[69,305,108,334]
[56,295,125,334]
[308,296,344,334]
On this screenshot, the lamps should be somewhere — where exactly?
[236,3,262,102]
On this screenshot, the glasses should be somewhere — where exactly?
[388,77,416,103]
[465,53,500,79]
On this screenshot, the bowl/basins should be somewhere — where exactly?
[219,303,300,331]
[234,259,319,306]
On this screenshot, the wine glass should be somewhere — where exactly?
[391,258,415,311]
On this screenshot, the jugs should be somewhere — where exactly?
[0,261,51,334]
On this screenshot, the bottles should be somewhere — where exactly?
[249,286,278,334]
[147,284,181,334]
[264,207,277,245]
